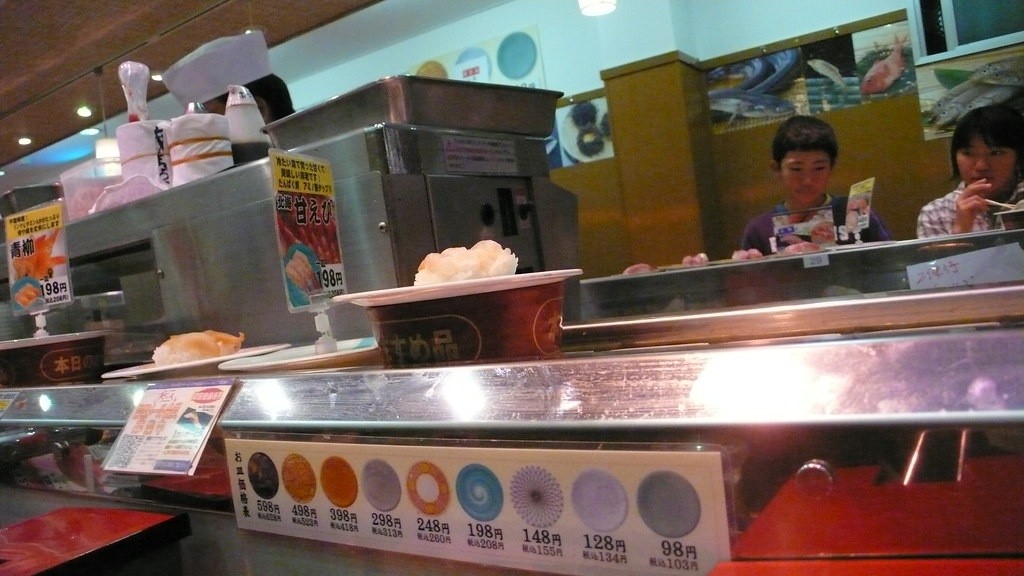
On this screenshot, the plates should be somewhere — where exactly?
[562,98,614,163]
[332,269,583,308]
[101,343,292,381]
[217,338,382,370]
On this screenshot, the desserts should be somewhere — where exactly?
[412,239,521,286]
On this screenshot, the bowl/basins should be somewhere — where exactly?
[993,209,1024,230]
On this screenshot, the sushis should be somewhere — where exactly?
[286,250,321,294]
[152,329,244,366]
[624,242,820,276]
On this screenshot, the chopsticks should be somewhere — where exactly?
[954,189,1016,209]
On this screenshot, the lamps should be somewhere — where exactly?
[578,0,617,15]
[96,66,118,158]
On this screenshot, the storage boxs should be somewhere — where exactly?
[60,155,122,220]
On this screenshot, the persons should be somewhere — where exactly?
[915,103,1024,239]
[742,116,890,254]
[161,32,296,125]
